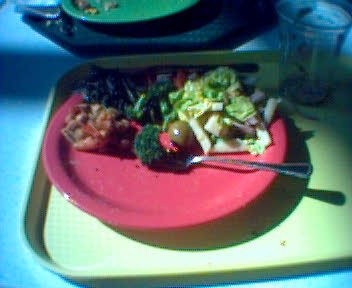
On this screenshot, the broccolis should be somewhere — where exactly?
[126,79,172,120]
[133,124,163,166]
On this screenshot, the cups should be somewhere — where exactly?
[277,1,351,105]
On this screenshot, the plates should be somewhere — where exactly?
[59,0,198,23]
[17,50,352,285]
[22,0,275,59]
[42,66,289,232]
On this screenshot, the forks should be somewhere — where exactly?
[148,152,314,182]
